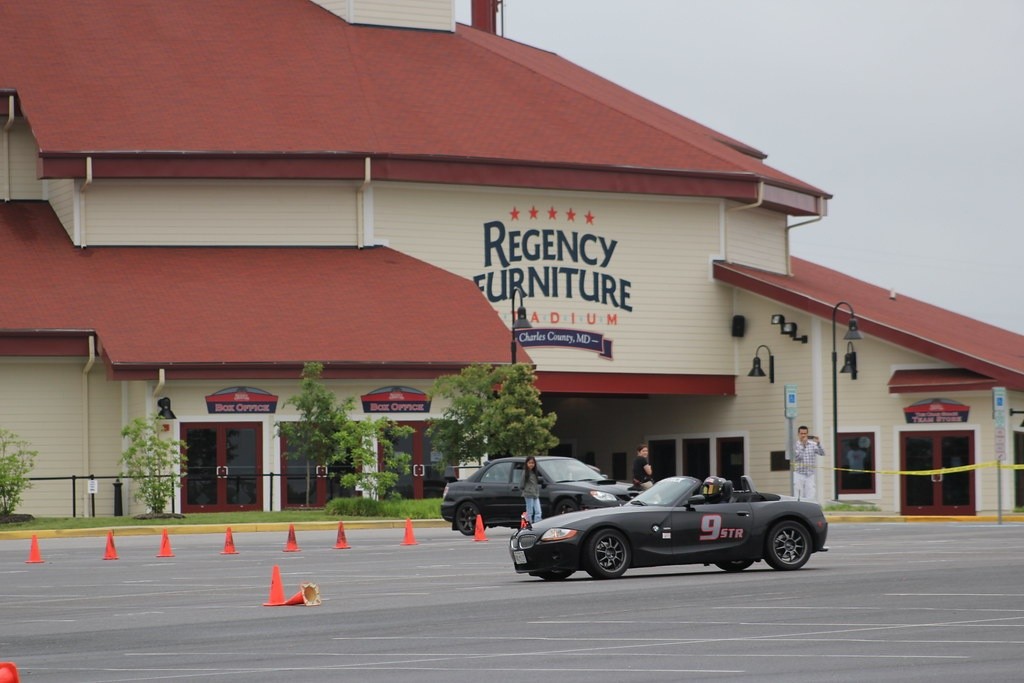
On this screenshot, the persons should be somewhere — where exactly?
[793,426,825,500]
[700,476,727,504]
[633,444,654,490]
[518,456,542,524]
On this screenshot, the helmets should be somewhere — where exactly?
[702,476,726,503]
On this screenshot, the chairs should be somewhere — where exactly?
[738,476,760,503]
[722,480,735,496]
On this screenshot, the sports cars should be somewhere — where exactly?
[508,474,828,583]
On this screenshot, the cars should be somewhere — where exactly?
[440,455,643,537]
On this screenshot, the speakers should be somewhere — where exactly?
[732,316,745,337]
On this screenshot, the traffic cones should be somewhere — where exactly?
[156,528,175,557]
[0,660,22,683]
[102,532,119,561]
[283,581,322,607]
[400,518,419,546]
[332,522,352,549]
[283,524,302,552]
[220,527,240,554]
[25,535,45,563]
[262,565,287,606]
[521,512,529,529]
[470,514,488,541]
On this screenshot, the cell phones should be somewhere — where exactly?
[807,436,815,439]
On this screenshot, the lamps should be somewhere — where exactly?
[156,397,177,419]
[748,344,775,383]
[839,342,857,380]
[769,315,807,343]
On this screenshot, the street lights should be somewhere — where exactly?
[509,285,533,364]
[831,300,863,500]
